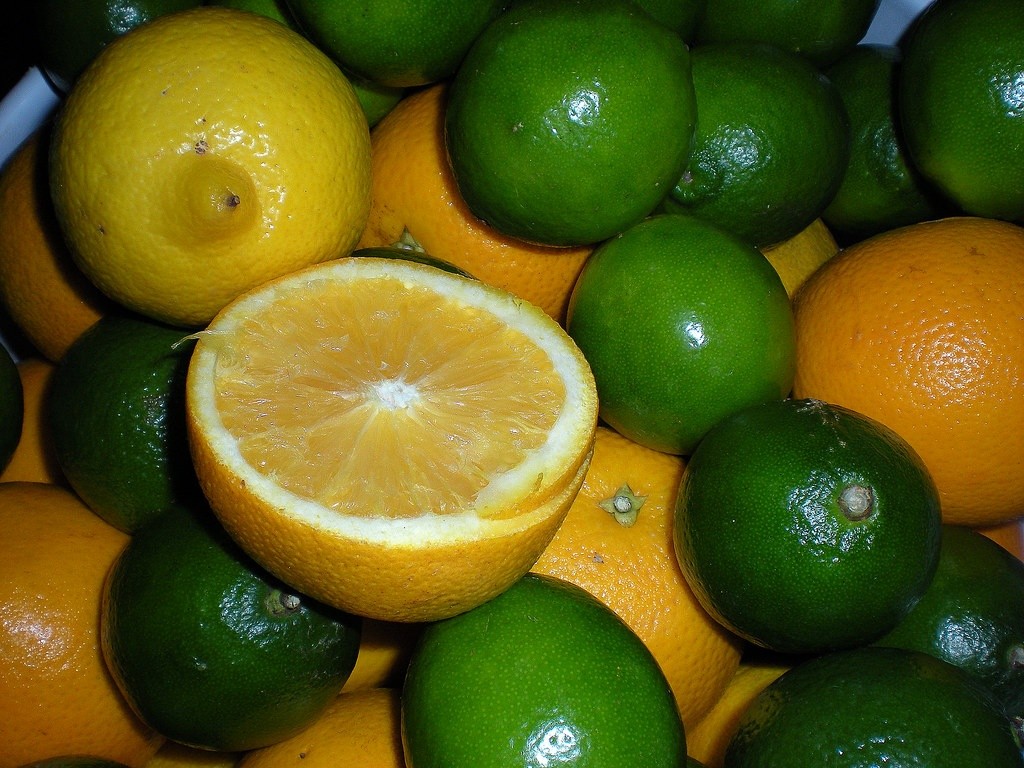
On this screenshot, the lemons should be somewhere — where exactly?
[0,0,1024,768]
